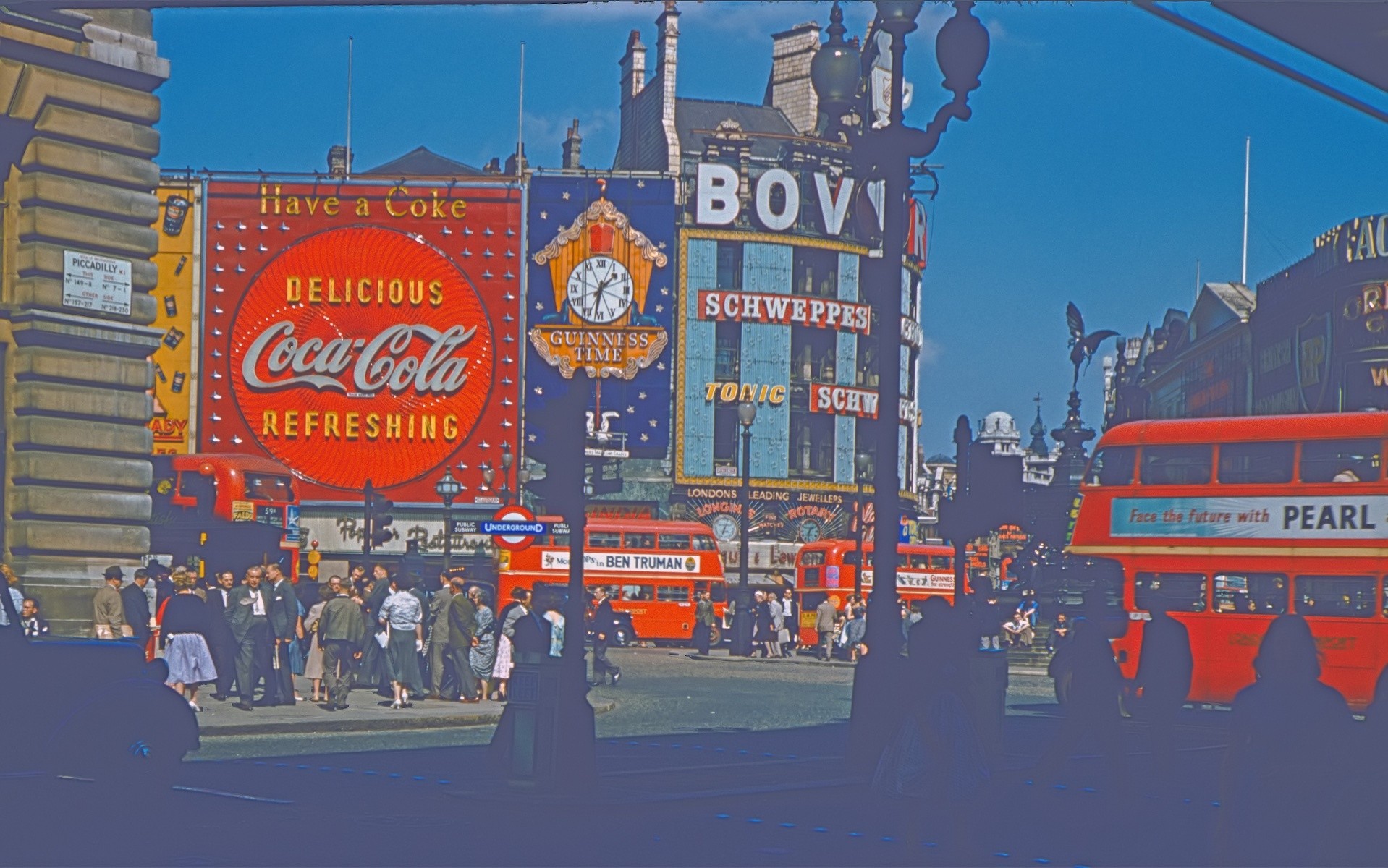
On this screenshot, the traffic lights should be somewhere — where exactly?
[372,493,394,549]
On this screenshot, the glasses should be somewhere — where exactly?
[23,606,33,610]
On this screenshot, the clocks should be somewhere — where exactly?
[566,255,634,324]
[799,519,821,544]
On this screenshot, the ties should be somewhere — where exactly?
[239,592,260,612]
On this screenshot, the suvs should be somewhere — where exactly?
[0,569,145,736]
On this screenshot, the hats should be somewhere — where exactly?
[101,565,125,577]
[755,591,763,596]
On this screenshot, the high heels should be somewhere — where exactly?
[390,699,401,710]
[498,691,504,702]
[402,692,408,705]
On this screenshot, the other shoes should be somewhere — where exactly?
[309,698,319,702]
[611,672,621,685]
[751,651,783,659]
[320,704,334,711]
[324,700,328,702]
[336,704,349,709]
[209,693,226,701]
[592,681,605,686]
[232,701,253,711]
[423,694,441,700]
[295,697,304,701]
[463,697,479,703]
[817,656,822,661]
[826,658,830,661]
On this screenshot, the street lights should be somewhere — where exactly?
[807,0,990,732]
[484,447,538,513]
[853,447,870,605]
[729,394,756,657]
[433,464,465,580]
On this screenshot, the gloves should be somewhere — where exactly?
[416,639,423,653]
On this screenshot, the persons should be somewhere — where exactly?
[638,592,650,647]
[1217,577,1287,614]
[624,533,654,548]
[1217,614,1358,867]
[0,563,50,637]
[691,590,715,655]
[1301,595,1352,617]
[1049,589,1193,807]
[850,595,1008,721]
[833,593,922,662]
[1331,456,1359,482]
[752,587,797,657]
[93,566,127,639]
[1047,612,1068,654]
[967,568,994,595]
[121,568,152,648]
[977,595,1002,649]
[1002,594,1038,648]
[148,556,622,717]
[622,590,629,600]
[814,596,838,661]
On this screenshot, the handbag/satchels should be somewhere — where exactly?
[777,628,791,644]
[374,620,390,649]
[307,618,319,635]
[421,625,433,658]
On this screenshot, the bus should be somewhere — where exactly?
[791,540,985,648]
[150,451,310,587]
[1059,410,1386,726]
[495,515,732,649]
